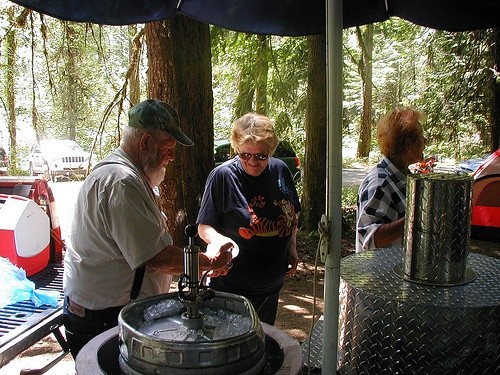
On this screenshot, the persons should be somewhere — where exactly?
[63,99,234,362]
[196,114,301,326]
[355,107,427,252]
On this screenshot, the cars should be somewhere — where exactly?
[213,138,302,189]
[26,139,96,181]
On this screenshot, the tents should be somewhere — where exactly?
[471,149,500,241]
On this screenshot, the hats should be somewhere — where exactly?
[127,99,195,146]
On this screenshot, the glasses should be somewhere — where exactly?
[237,149,268,160]
